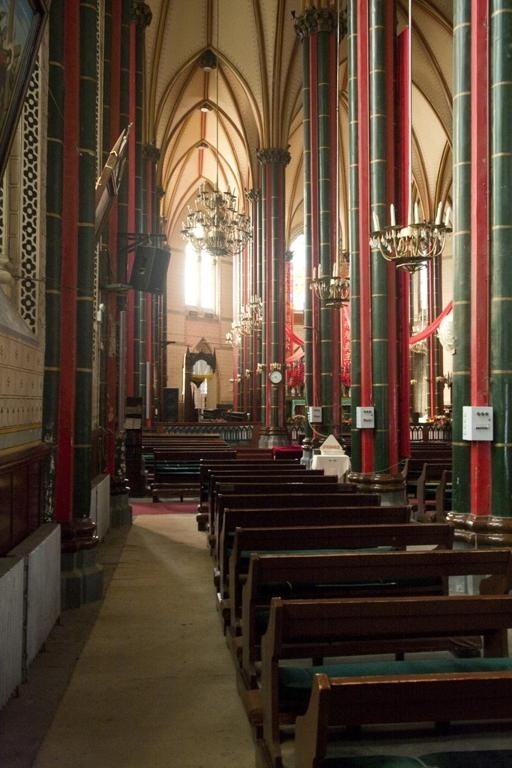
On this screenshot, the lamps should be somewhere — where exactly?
[180,0,252,263]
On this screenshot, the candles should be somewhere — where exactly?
[312,263,336,280]
[374,201,451,232]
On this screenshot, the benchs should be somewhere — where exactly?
[141,435,238,503]
[197,443,512,768]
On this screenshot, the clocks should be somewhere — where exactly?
[269,370,283,384]
